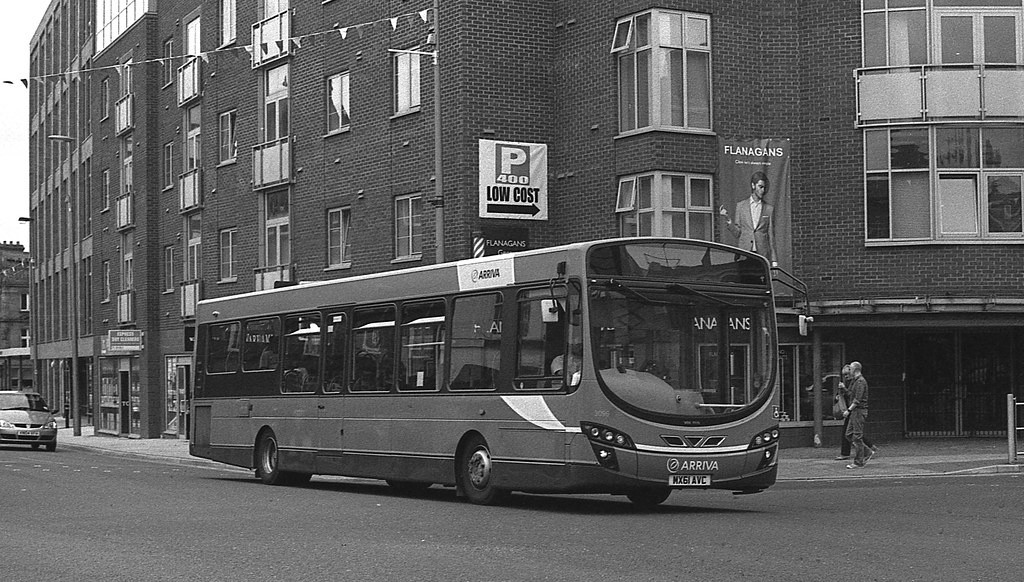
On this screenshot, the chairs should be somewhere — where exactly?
[300,372,316,392]
[283,370,301,392]
[352,350,406,391]
[328,374,343,392]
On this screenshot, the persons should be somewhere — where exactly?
[835,365,878,460]
[720,171,778,274]
[259,336,278,370]
[550,326,583,387]
[839,362,874,468]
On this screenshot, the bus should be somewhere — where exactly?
[185,233,816,505]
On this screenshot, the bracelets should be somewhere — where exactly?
[847,409,852,412]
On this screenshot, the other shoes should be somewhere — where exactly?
[845,463,864,469]
[870,445,879,451]
[862,450,874,463]
[835,455,850,460]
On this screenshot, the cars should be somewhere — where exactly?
[0,390,59,453]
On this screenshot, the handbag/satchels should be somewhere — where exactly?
[832,385,847,419]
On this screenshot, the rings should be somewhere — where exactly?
[845,415,846,416]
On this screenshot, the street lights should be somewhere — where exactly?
[47,134,83,438]
[19,216,38,394]
